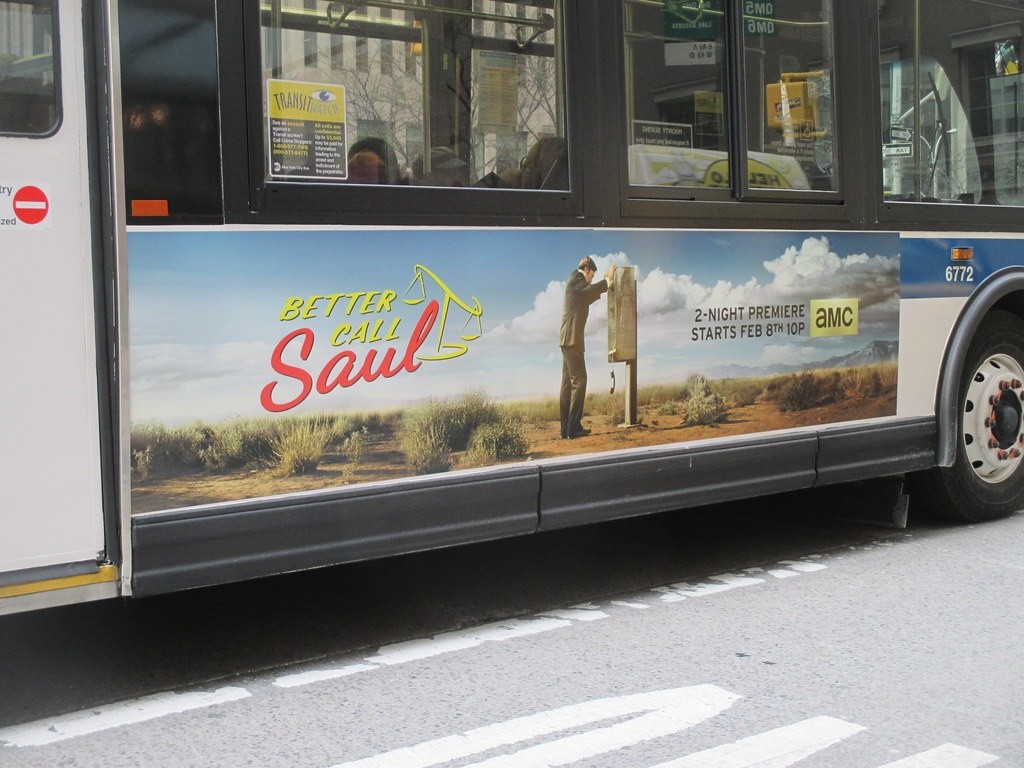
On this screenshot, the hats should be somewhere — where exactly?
[411,146,467,178]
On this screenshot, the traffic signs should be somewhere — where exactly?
[882,127,915,160]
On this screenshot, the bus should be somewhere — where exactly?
[0,0,1024,615]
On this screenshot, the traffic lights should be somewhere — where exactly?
[411,18,422,55]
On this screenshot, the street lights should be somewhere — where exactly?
[919,129,959,198]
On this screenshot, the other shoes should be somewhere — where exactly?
[562,429,592,439]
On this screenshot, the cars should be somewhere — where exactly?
[995,40,1013,74]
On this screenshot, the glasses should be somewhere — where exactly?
[519,157,533,171]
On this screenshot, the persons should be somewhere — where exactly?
[348,136,567,191]
[559,256,618,440]
